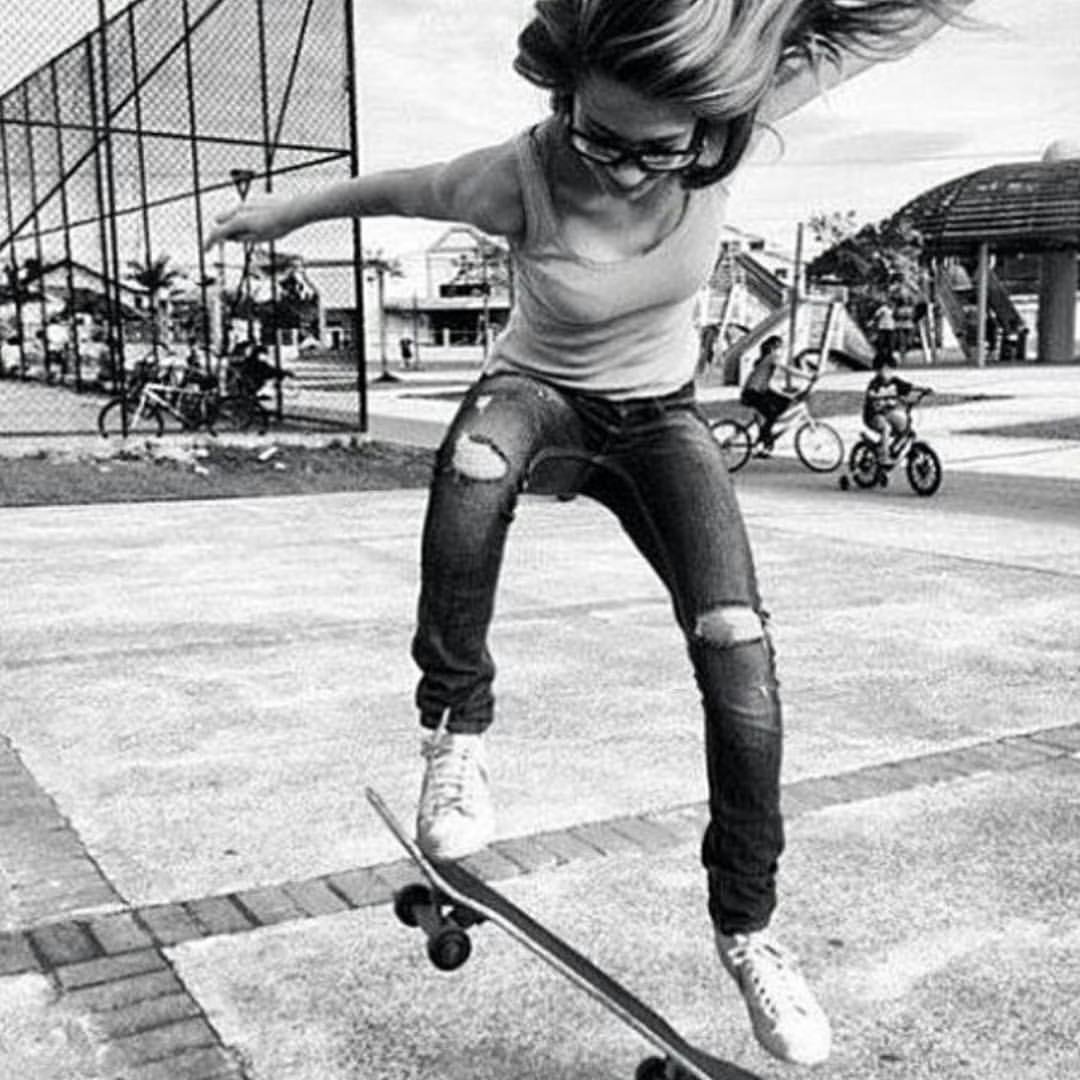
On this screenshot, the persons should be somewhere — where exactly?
[200,0,978,1065]
[6,312,237,404]
[738,334,814,458]
[860,287,1030,367]
[863,349,934,471]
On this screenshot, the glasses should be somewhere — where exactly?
[560,98,708,175]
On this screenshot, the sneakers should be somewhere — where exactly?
[416,730,495,869]
[715,925,833,1067]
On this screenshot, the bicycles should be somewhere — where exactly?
[95,361,271,438]
[711,374,845,475]
[849,386,944,499]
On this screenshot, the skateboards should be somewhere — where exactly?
[357,777,776,1080]
